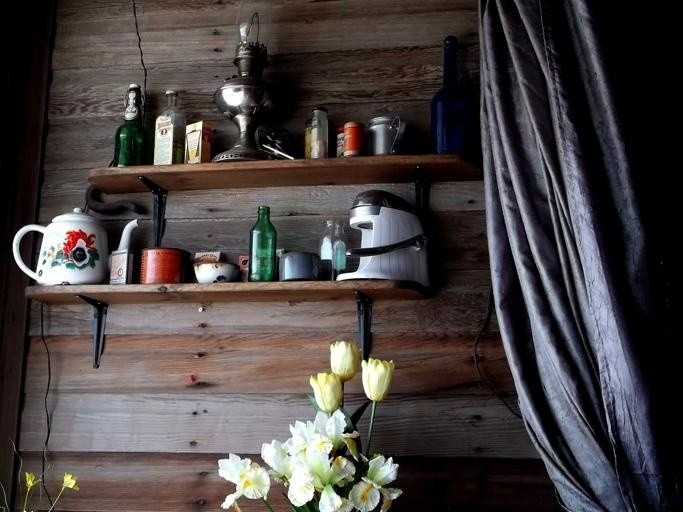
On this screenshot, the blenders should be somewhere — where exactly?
[336,190,430,287]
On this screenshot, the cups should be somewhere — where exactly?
[366,115,407,154]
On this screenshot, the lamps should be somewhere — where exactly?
[211,0,277,162]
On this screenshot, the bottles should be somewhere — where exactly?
[112,80,151,168]
[304,107,330,161]
[320,219,347,281]
[153,88,188,167]
[429,34,475,156]
[250,205,277,281]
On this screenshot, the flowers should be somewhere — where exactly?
[21,473,80,512]
[217,341,404,512]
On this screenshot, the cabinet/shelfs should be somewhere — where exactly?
[24,154,471,369]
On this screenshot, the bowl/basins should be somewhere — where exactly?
[193,260,241,283]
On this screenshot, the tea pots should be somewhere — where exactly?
[12,206,139,287]
